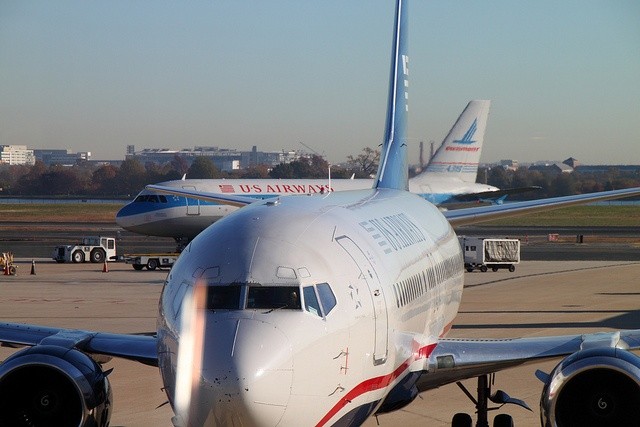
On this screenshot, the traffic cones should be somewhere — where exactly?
[103,259,109,272]
[31,259,36,273]
[4,259,9,274]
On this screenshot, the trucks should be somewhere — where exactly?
[52,236,116,262]
[460,239,521,272]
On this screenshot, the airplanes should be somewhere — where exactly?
[1,0,640,426]
[115,100,543,252]
